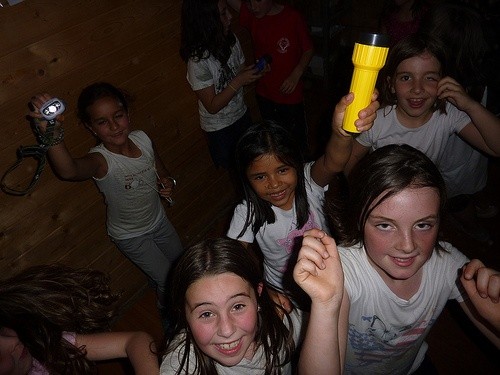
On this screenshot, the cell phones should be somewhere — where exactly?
[248,54,272,75]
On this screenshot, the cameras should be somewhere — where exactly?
[40,98,65,121]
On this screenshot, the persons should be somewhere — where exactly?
[293,143,500,375]
[345,1,500,242]
[180,0,274,198]
[28,83,186,309]
[225,86,381,322]
[238,0,317,157]
[158,236,308,375]
[376,0,426,39]
[0,264,158,374]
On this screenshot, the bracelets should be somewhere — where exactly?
[228,83,239,94]
[38,129,67,147]
[168,176,177,186]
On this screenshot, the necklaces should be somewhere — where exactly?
[99,136,173,204]
